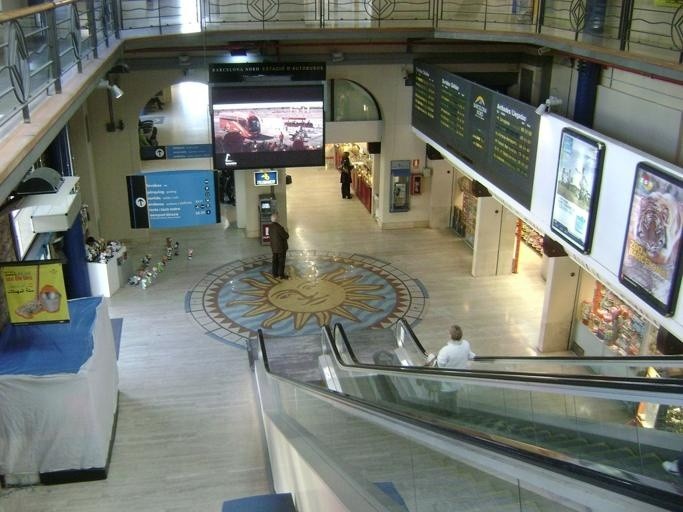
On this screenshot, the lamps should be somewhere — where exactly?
[94,79,128,103]
[533,95,563,119]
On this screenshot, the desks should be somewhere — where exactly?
[335,142,373,214]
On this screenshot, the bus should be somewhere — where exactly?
[220,115,260,140]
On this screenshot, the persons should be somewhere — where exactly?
[560,165,589,200]
[268,213,289,281]
[421,323,476,421]
[339,151,354,199]
[217,132,307,152]
[138,120,158,147]
[244,110,261,136]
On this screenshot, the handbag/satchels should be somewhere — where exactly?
[417,356,440,392]
[338,160,349,173]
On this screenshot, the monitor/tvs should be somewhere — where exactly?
[254,171,278,186]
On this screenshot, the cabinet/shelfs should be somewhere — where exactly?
[572,277,660,419]
[457,176,546,274]
[87,243,133,298]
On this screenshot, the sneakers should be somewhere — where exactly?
[273,274,288,278]
